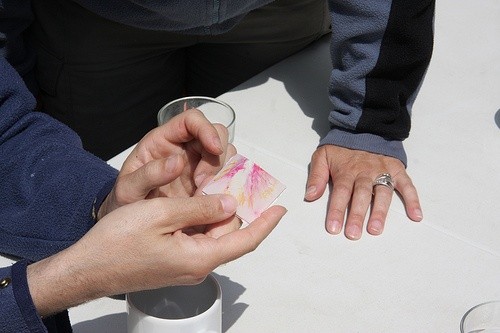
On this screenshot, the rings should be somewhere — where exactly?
[372,172,395,195]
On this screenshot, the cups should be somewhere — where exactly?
[124,273,221,333]
[159,95,237,160]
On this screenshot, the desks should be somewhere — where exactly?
[0,1,499,333]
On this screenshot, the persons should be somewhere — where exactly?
[0,51,289,333]
[14,0,438,241]
